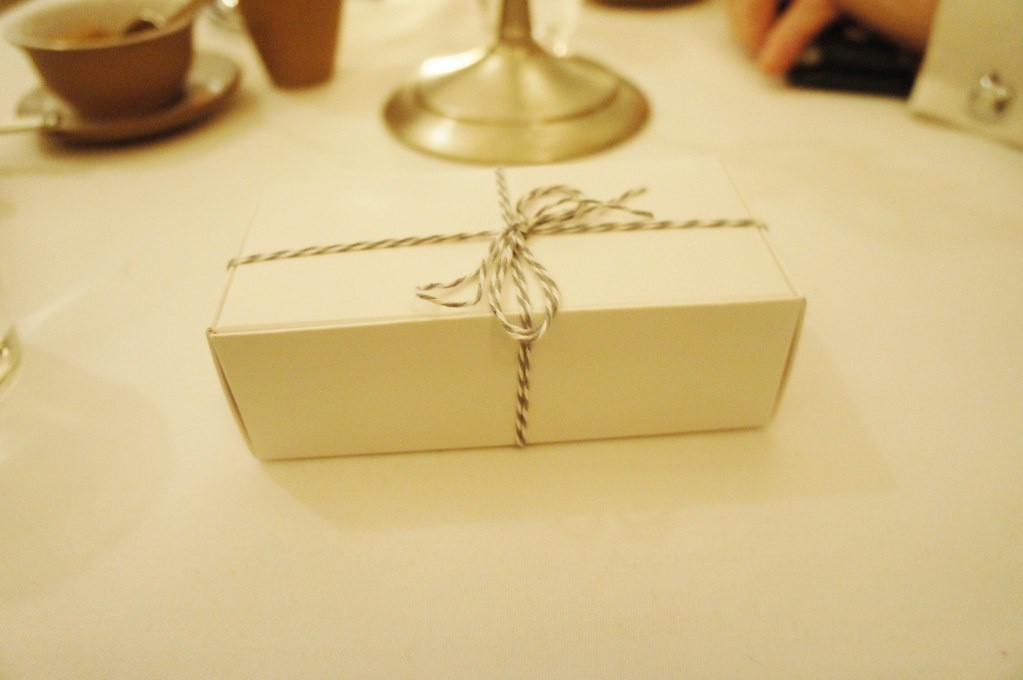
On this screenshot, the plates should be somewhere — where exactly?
[12,47,240,142]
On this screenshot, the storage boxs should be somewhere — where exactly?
[201,156,807,461]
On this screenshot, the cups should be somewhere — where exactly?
[206,0,341,90]
[0,0,203,115]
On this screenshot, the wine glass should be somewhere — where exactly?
[383,0,649,165]
